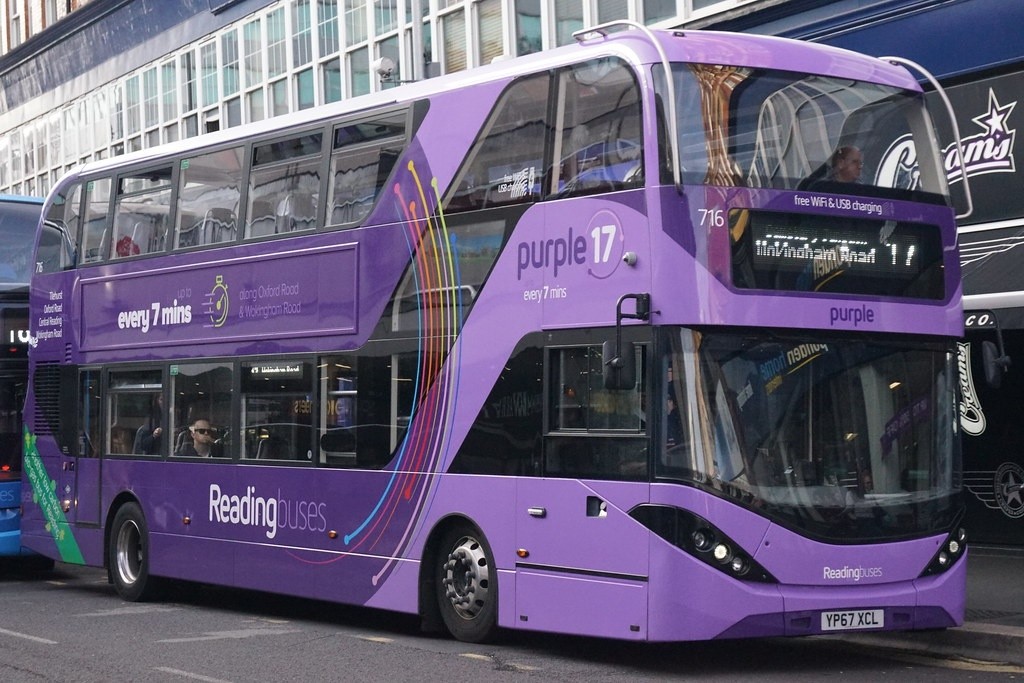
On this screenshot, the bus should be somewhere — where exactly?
[17,19,1011,644]
[0,194,47,559]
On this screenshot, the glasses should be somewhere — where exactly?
[194,429,212,435]
[840,158,865,169]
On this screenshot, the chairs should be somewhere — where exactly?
[280,193,318,233]
[250,201,277,237]
[132,425,146,454]
[201,208,237,244]
[255,437,291,460]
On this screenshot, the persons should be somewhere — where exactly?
[138,390,163,455]
[844,470,881,526]
[175,417,224,457]
[807,144,866,194]
